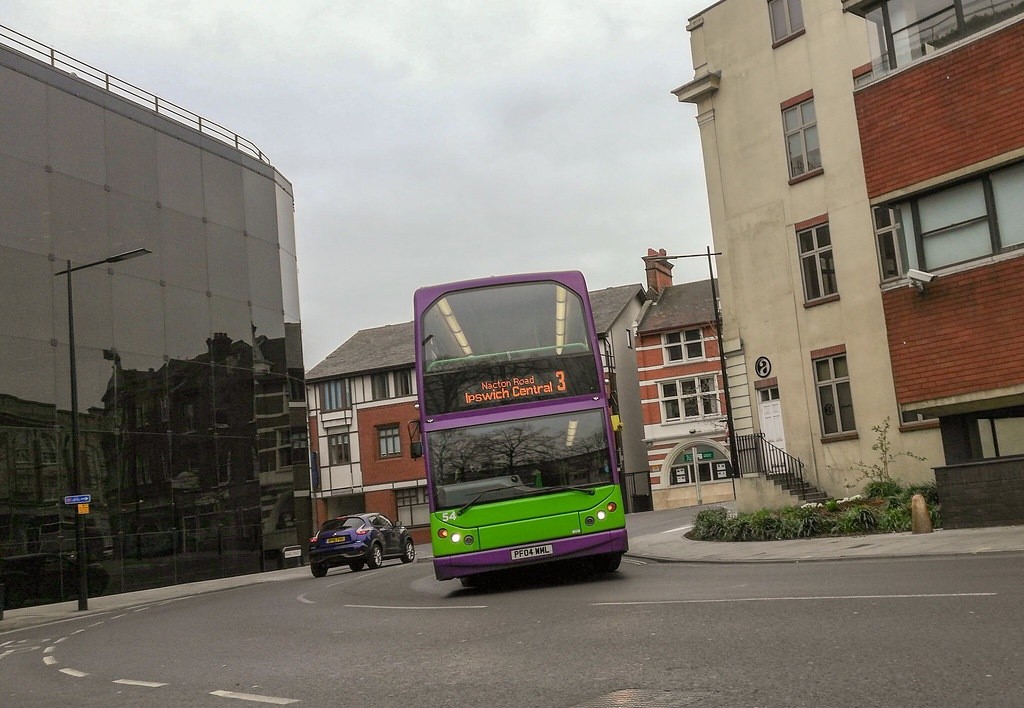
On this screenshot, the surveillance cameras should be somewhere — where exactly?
[908,269,938,283]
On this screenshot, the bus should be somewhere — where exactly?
[407,269,630,589]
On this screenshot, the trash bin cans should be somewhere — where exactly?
[282,546,301,568]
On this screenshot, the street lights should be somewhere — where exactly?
[55,247,153,611]
[645,245,741,480]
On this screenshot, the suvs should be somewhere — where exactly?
[0,552,110,612]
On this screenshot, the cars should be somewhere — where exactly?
[308,512,416,578]
[1,498,301,568]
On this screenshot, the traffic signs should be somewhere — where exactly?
[64,494,91,505]
